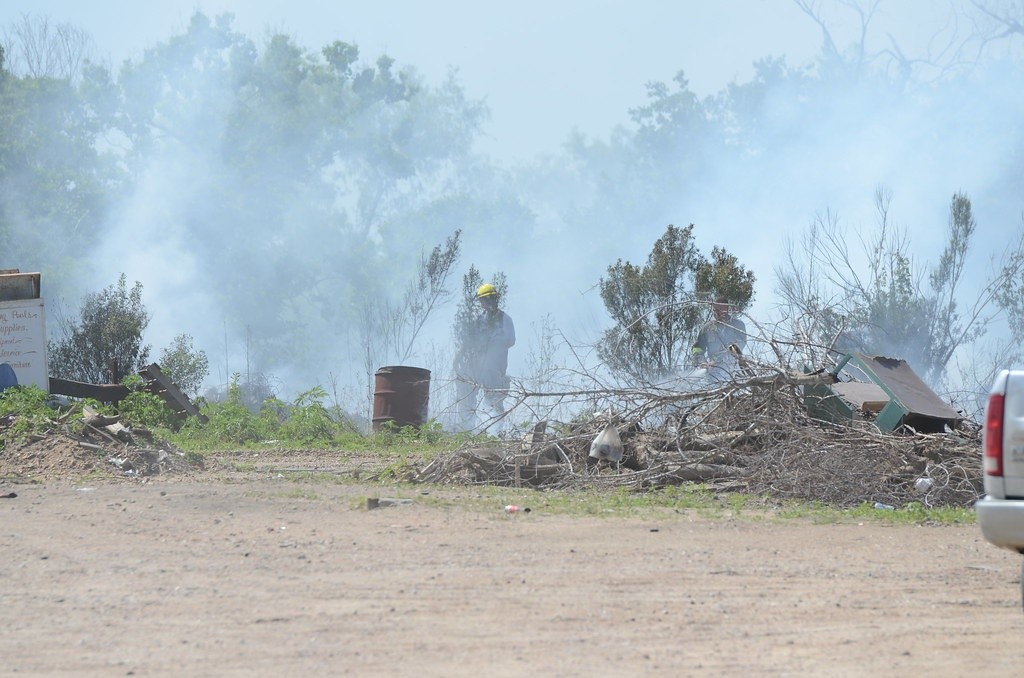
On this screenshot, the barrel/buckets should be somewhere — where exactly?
[372,366,431,434]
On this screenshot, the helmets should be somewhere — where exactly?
[475,284,497,301]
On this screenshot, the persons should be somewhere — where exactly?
[453,284,515,436]
[692,297,747,391]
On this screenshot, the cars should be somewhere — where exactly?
[975,370,1024,554]
